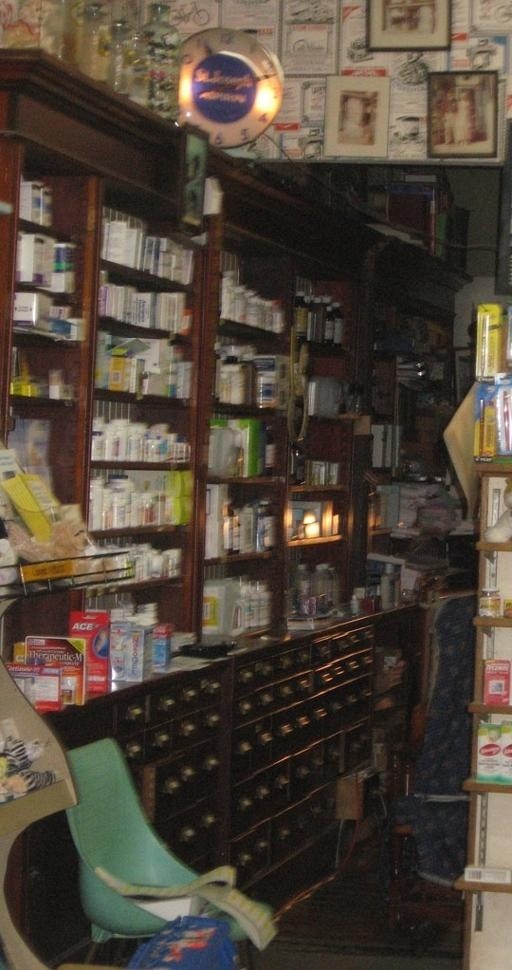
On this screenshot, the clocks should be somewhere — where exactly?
[176,27,284,150]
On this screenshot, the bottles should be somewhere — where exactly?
[84,289,401,669]
[78,2,181,122]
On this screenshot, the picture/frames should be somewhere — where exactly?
[366,0,452,51]
